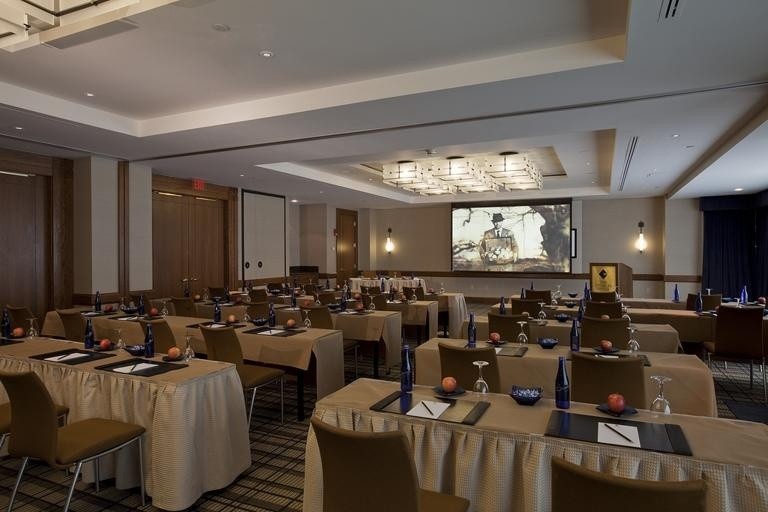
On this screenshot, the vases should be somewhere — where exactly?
[184,289,189,297]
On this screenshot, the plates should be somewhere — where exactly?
[147,314,164,316]
[122,307,137,314]
[104,310,117,312]
[94,341,115,351]
[122,346,144,356]
[283,327,298,329]
[595,403,638,417]
[710,309,716,313]
[162,354,184,361]
[7,335,26,339]
[528,317,534,320]
[355,308,365,312]
[485,340,507,345]
[334,288,341,291]
[434,396,457,408]
[432,385,466,397]
[400,300,407,302]
[427,292,436,294]
[353,297,360,301]
[594,347,619,353]
[226,322,239,323]
[194,299,203,303]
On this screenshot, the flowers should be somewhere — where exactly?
[176,272,200,289]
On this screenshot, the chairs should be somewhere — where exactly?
[56,309,87,342]
[360,285,387,311]
[0,371,146,511]
[686,294,722,311]
[247,288,272,303]
[583,302,622,319]
[512,298,543,319]
[170,296,197,318]
[403,287,424,300]
[581,317,630,350]
[138,318,176,353]
[0,401,70,477]
[129,293,153,315]
[267,282,282,294]
[311,417,470,512]
[304,284,317,302]
[319,293,337,305]
[488,312,529,343]
[438,343,500,393]
[6,304,39,337]
[208,287,230,303]
[590,291,615,302]
[552,456,708,512]
[525,289,551,305]
[198,323,285,430]
[571,352,645,409]
[310,306,333,329]
[318,278,336,289]
[703,304,763,389]
[246,302,270,320]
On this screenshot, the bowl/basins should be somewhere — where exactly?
[272,290,280,295]
[538,339,558,349]
[251,321,267,326]
[565,303,576,308]
[508,385,543,406]
[568,294,577,298]
[555,317,570,322]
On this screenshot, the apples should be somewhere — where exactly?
[429,288,433,292]
[104,305,113,313]
[354,293,362,299]
[285,319,296,330]
[607,392,625,412]
[490,333,501,342]
[600,339,613,351]
[193,295,202,300]
[166,346,180,360]
[601,315,610,320]
[303,300,312,307]
[757,296,766,304]
[228,315,235,322]
[99,339,110,350]
[336,285,341,289]
[354,302,364,309]
[442,377,457,392]
[149,308,158,316]
[13,327,24,337]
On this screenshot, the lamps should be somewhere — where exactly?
[636,222,647,253]
[382,149,543,197]
[385,228,394,254]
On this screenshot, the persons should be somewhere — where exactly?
[479,213,519,263]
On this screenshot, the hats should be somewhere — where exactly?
[491,213,503,222]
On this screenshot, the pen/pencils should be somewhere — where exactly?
[130,363,137,372]
[57,354,69,359]
[605,424,634,444]
[422,401,434,416]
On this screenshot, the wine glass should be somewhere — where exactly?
[473,361,489,400]
[626,327,641,355]
[203,288,208,301]
[161,301,168,317]
[618,294,624,307]
[25,318,37,343]
[551,294,558,307]
[613,286,619,300]
[650,376,672,419]
[240,308,250,326]
[555,283,563,297]
[734,298,742,309]
[303,310,312,330]
[706,289,712,295]
[411,289,418,301]
[300,284,306,295]
[621,306,631,326]
[365,287,370,295]
[119,297,126,313]
[185,336,196,361]
[538,303,547,320]
[368,296,376,313]
[343,280,347,290]
[315,293,321,306]
[516,321,528,348]
[440,282,445,294]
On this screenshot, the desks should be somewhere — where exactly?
[349,277,426,291]
[508,295,687,309]
[1,336,252,511]
[415,338,718,417]
[303,377,767,511]
[490,303,716,342]
[42,311,344,421]
[194,299,402,375]
[228,292,438,346]
[313,290,468,338]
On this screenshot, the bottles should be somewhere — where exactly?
[85,319,93,349]
[144,324,155,357]
[500,297,506,315]
[388,285,394,302]
[224,287,230,303]
[531,279,533,291]
[468,343,476,348]
[214,299,221,322]
[184,286,188,298]
[468,312,476,343]
[675,284,679,301]
[578,308,582,323]
[95,291,101,312]
[0,308,12,337]
[581,307,585,321]
[326,280,329,289]
[138,294,145,315]
[585,289,590,306]
[291,288,296,307]
[741,285,748,304]
[579,299,583,308]
[381,279,384,292]
[400,393,412,414]
[418,280,421,287]
[269,303,275,327]
[584,282,587,299]
[696,291,702,312]
[570,317,579,351]
[521,288,524,299]
[558,411,570,437]
[401,345,412,391]
[555,356,569,409]
[341,295,346,312]
